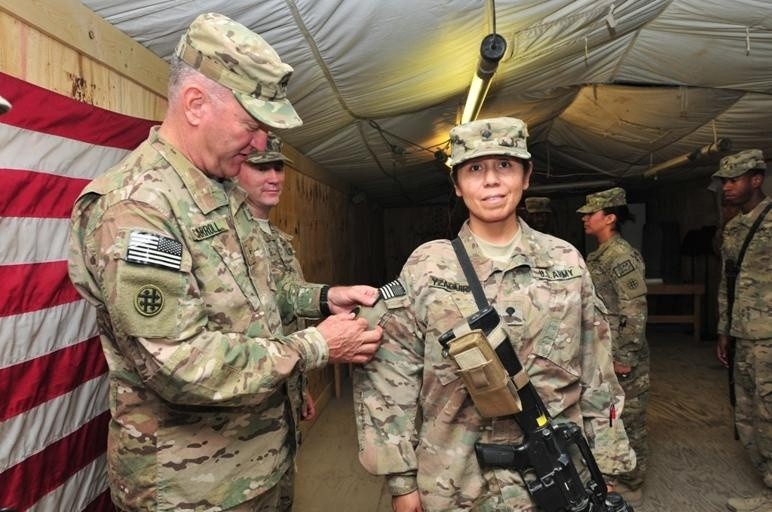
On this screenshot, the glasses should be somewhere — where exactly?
[434,149,452,170]
[455,32,507,127]
[642,119,732,180]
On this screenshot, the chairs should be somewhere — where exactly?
[728,490,772,512]
[613,481,644,507]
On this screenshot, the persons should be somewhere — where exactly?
[345,116,638,511]
[710,147,771,512]
[234,127,318,474]
[66,8,384,511]
[523,196,552,231]
[576,187,651,508]
[711,195,740,258]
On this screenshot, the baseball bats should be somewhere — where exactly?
[441,306,634,512]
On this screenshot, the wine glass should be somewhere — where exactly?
[622,374,627,378]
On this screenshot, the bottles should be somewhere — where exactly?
[576,185,627,214]
[175,13,303,129]
[244,130,295,168]
[450,117,532,166]
[712,149,768,179]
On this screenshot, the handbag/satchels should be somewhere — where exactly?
[319,282,330,318]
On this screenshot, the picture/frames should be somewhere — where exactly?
[646,283,706,340]
